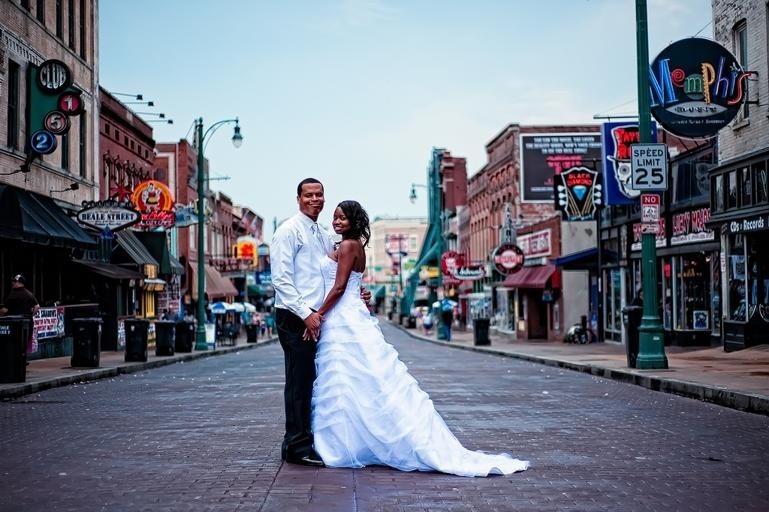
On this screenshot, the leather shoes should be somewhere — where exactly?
[286,453,324,466]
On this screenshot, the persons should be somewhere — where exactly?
[301,200,532,479]
[268,177,372,467]
[0,274,41,367]
[419,286,644,342]
[160,307,273,347]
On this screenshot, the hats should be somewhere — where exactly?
[12,275,24,282]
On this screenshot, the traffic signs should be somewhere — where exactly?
[625,143,670,192]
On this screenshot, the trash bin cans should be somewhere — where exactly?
[622,305,643,369]
[71,317,104,368]
[0,314,31,384]
[472,318,491,346]
[174,321,194,353]
[123,319,150,362]
[245,323,258,343]
[154,320,175,356]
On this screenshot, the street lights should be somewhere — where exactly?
[408,181,449,340]
[190,116,246,349]
[383,232,405,292]
